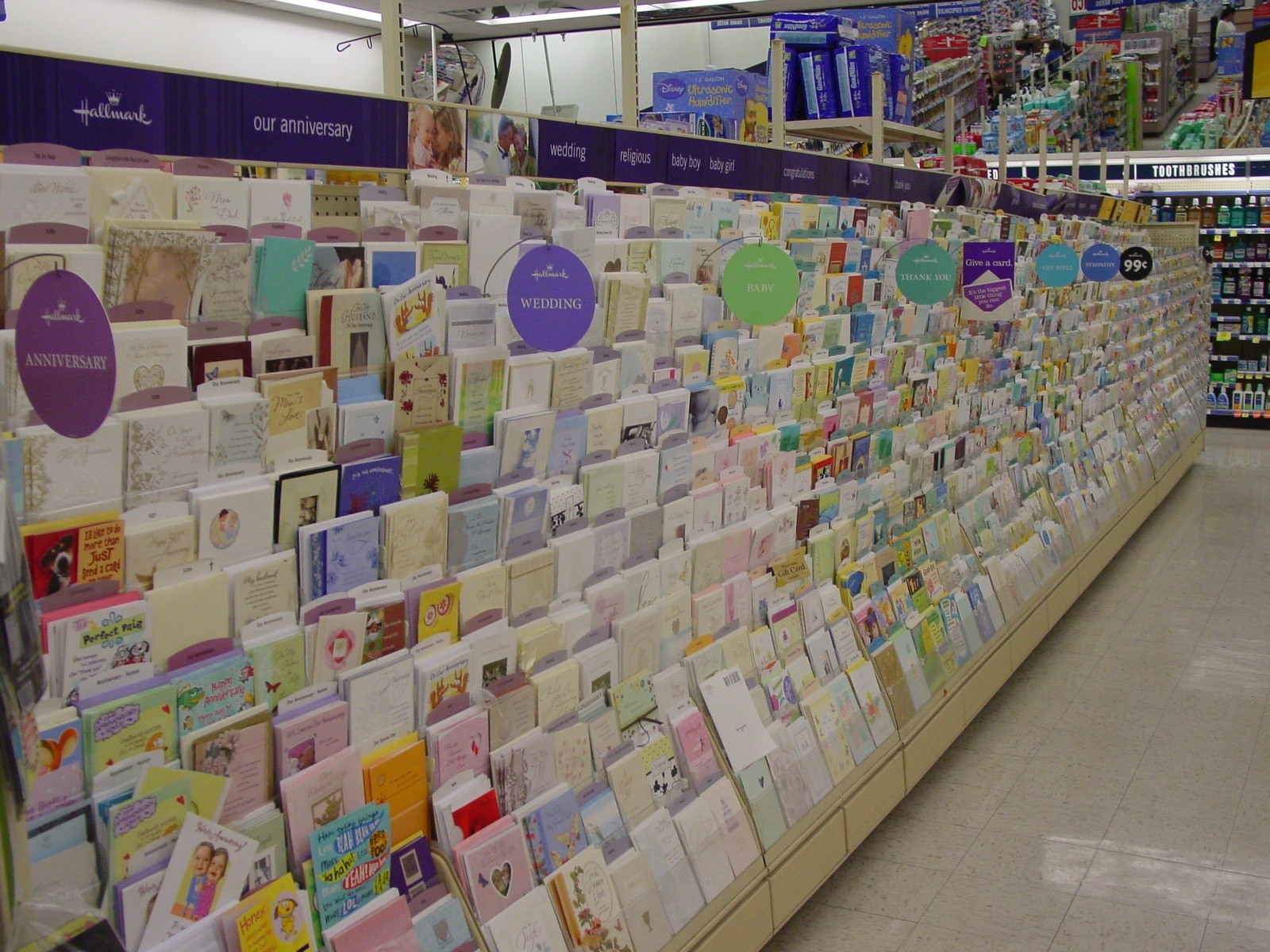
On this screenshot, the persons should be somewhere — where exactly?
[412,103,435,169]
[1213,6,1238,57]
[510,122,536,177]
[483,113,514,177]
[427,107,466,173]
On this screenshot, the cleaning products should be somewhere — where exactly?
[1206,382,1270,410]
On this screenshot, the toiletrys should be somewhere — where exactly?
[1147,195,1270,229]
[1212,267,1270,299]
[1241,306,1268,335]
[1199,235,1270,264]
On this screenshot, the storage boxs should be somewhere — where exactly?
[606,12,914,138]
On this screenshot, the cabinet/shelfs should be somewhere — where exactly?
[0,32,1270,952]
[418,48,476,102]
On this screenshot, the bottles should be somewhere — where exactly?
[1257,234,1267,262]
[1160,197,1175,221]
[1207,387,1216,408]
[1217,386,1229,409]
[1231,197,1244,228]
[1260,196,1270,228]
[1238,276,1251,297]
[1201,196,1217,228]
[1217,199,1231,228]
[1254,307,1268,335]
[1225,241,1234,261]
[1234,235,1246,262]
[1245,196,1259,227]
[1246,241,1256,262]
[1175,198,1188,221]
[1222,277,1236,298]
[1241,307,1254,335]
[1188,198,1201,228]
[1251,268,1267,298]
[1213,242,1224,262]
[1143,106,1159,122]
[1175,8,1185,25]
[1232,383,1270,410]
[1150,199,1160,221]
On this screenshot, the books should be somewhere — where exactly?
[0,163,1209,952]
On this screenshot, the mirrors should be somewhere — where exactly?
[411,44,486,106]
